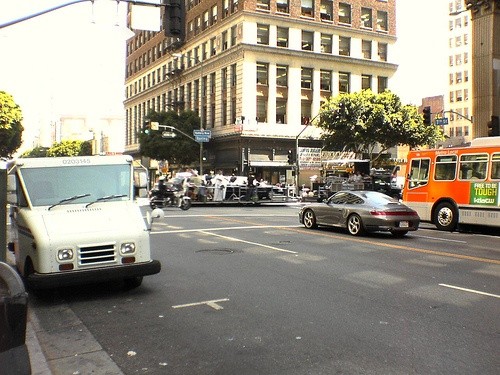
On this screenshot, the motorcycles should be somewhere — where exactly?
[149,183,192,211]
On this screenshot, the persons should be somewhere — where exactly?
[472,162,483,178]
[422,171,428,179]
[347,170,372,182]
[277,119,282,124]
[184,170,266,202]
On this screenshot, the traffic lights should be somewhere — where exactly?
[423,106,431,126]
[487,115,500,137]
[143,119,151,135]
[287,148,294,165]
[338,101,346,118]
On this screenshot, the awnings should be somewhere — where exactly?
[249,161,295,166]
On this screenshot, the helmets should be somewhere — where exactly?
[159,176,165,180]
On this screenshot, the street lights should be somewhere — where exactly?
[171,53,204,175]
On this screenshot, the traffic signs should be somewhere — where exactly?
[161,130,176,139]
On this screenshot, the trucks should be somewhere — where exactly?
[6,153,164,292]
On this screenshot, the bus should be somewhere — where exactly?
[400,136,500,232]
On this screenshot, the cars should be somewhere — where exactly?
[298,190,420,237]
[224,175,285,201]
